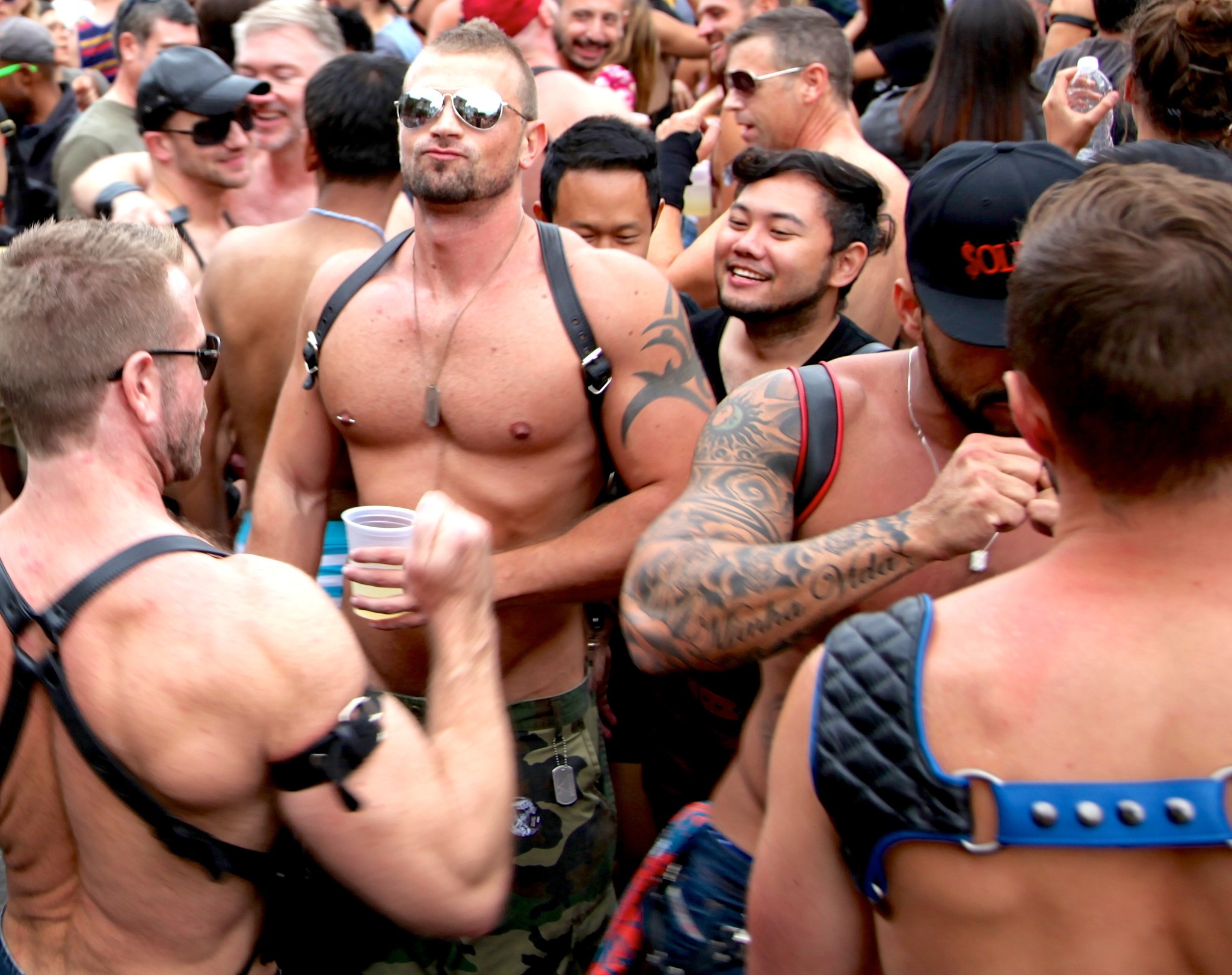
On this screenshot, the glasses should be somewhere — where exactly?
[153,106,254,146]
[109,332,219,381]
[727,67,834,96]
[394,88,532,131]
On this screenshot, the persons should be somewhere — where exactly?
[0,1,1230,975]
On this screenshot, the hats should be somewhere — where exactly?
[137,44,270,135]
[905,141,1087,348]
[463,0,543,39]
[1,15,55,63]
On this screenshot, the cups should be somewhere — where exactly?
[341,504,418,623]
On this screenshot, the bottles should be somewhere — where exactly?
[1065,55,1114,165]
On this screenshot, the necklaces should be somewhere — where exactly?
[411,215,525,427]
[907,347,999,572]
[308,207,387,241]
